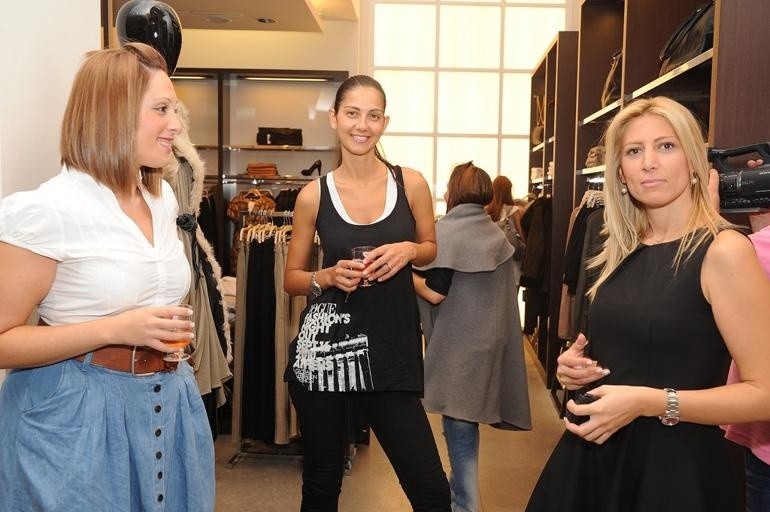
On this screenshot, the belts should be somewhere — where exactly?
[35,317,178,375]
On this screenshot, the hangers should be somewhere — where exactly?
[244,179,272,207]
[521,185,537,200]
[239,209,321,246]
[580,176,606,208]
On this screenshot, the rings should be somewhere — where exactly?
[561,382,566,392]
[386,262,394,272]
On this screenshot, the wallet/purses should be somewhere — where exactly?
[566,393,601,425]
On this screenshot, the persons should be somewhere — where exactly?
[283,74,452,512]
[483,175,527,294]
[2,38,219,510]
[411,160,535,512]
[703,157,770,512]
[522,96,770,510]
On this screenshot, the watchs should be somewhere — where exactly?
[658,386,682,429]
[308,272,322,298]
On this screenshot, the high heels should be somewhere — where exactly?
[301,159,321,176]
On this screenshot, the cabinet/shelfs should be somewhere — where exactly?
[528,31,578,389]
[168,68,348,276]
[572,0,770,209]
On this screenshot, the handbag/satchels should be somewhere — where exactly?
[507,231,525,260]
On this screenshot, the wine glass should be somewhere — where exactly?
[350,246,376,288]
[163,304,194,362]
